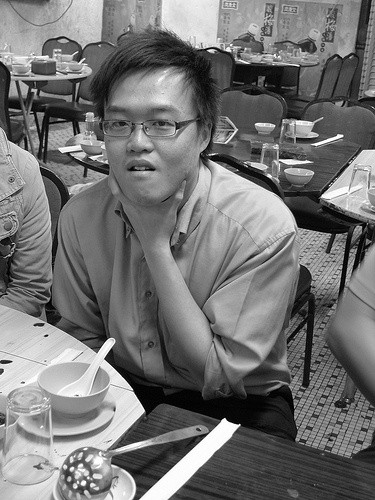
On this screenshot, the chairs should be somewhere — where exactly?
[0,31,375,387]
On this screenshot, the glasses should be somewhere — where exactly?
[99,117,200,138]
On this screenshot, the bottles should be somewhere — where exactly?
[83,112,97,140]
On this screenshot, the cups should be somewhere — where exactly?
[210,37,301,64]
[0,392,20,442]
[3,386,55,485]
[260,141,280,172]
[347,162,372,208]
[278,119,296,145]
[52,48,62,67]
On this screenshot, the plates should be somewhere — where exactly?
[10,72,31,77]
[63,69,84,73]
[286,132,319,137]
[16,376,116,438]
[52,465,136,500]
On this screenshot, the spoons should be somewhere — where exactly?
[55,337,117,396]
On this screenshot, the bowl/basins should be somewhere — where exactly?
[254,123,276,135]
[244,161,315,188]
[37,362,112,419]
[78,140,104,154]
[289,119,315,136]
[368,189,375,206]
[11,54,83,74]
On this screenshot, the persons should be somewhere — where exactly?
[1,124,52,323]
[323,244,374,463]
[56,28,297,444]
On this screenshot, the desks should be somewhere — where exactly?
[233,52,319,97]
[63,128,362,307]
[0,60,91,159]
[0,304,375,500]
